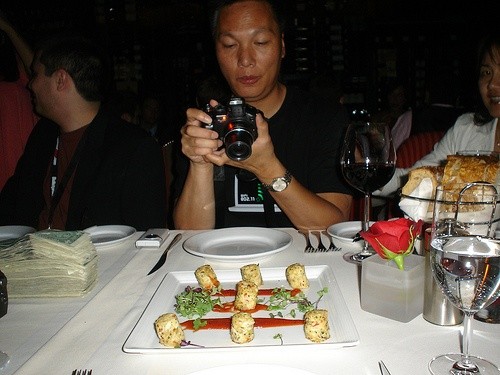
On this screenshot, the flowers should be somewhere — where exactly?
[358,216,425,272]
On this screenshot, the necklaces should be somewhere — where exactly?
[496,140,500,147]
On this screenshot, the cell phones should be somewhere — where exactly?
[135,229,170,248]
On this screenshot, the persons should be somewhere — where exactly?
[0,32,174,231]
[169,0,354,244]
[371,34,500,199]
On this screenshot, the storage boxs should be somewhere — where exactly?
[361,253,425,323]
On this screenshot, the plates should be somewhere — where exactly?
[325,220,376,243]
[0,225,37,241]
[121,264,360,355]
[83,224,136,246]
[182,227,293,263]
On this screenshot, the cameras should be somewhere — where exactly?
[202,95,264,161]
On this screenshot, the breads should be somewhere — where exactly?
[400,153,500,216]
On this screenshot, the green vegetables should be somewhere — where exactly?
[174,284,224,331]
[258,287,328,345]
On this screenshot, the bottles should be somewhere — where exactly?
[424,219,465,326]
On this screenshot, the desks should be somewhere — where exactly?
[0,227,500,375]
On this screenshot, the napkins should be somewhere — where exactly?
[398,178,433,223]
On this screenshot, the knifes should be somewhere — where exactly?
[146,233,182,275]
[379,360,390,375]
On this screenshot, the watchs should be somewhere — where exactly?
[261,170,293,194]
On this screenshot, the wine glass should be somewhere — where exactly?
[340,121,396,265]
[429,183,500,375]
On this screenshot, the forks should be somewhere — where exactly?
[72,369,92,375]
[298,228,342,253]
